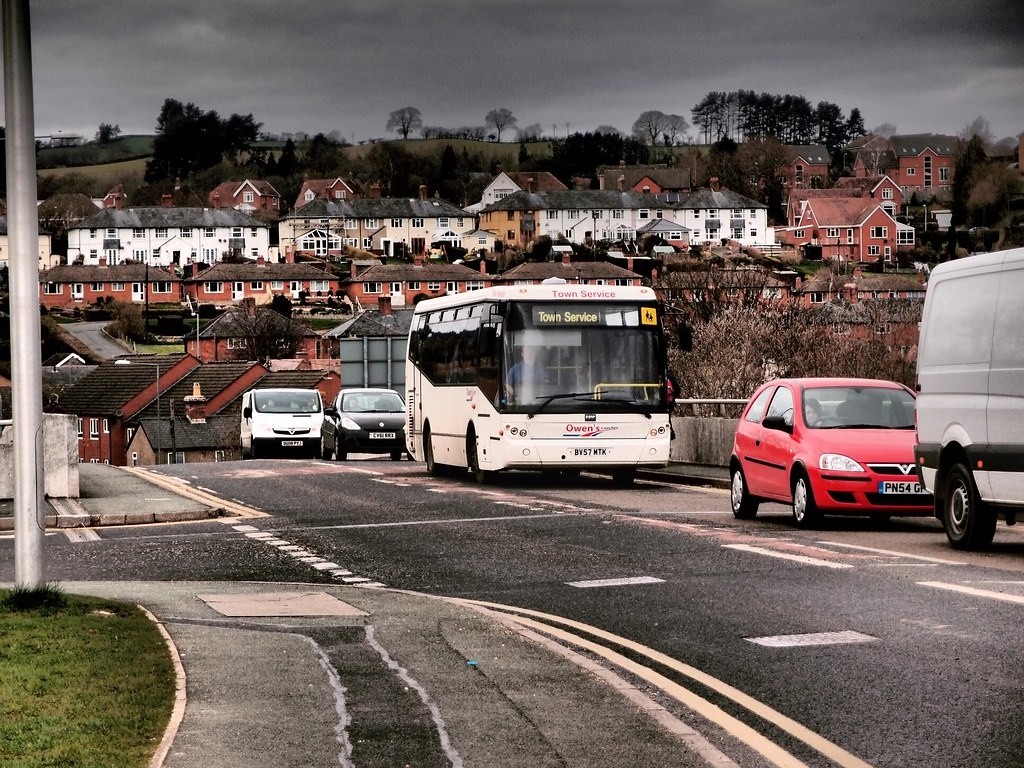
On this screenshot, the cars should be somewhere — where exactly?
[727,377,937,525]
[323,387,407,461]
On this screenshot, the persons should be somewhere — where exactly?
[504,346,549,403]
[344,397,360,411]
[804,398,823,427]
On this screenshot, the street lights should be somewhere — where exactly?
[113,358,160,467]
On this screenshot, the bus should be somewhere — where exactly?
[403,277,675,488]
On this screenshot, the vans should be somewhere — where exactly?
[916,245,1023,551]
[240,388,325,457]
